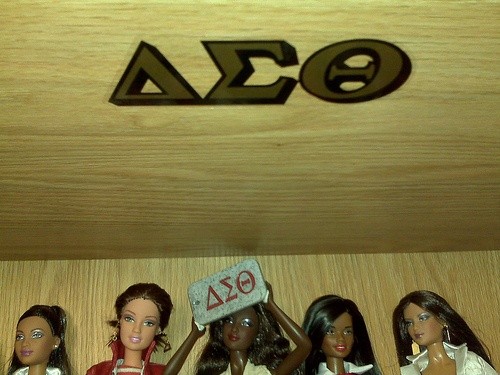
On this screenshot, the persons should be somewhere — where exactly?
[302,295,383,375]
[84,282,174,375]
[161,281,312,375]
[392,290,498,375]
[1,305,73,375]
